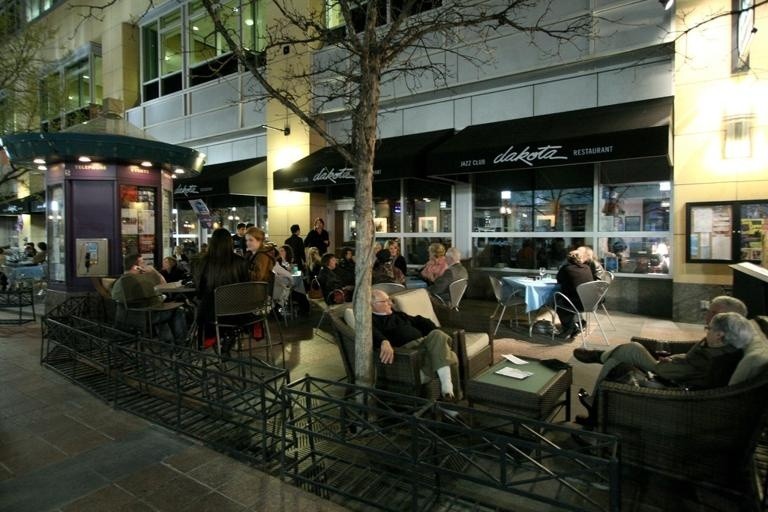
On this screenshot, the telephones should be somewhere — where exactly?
[85,253,91,272]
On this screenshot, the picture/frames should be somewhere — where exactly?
[418,216,438,232]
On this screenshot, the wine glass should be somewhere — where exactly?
[539,267,546,278]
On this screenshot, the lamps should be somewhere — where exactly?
[719,111,757,160]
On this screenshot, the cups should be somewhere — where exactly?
[546,274,551,281]
[535,276,541,281]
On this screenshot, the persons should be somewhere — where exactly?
[574,310,756,427]
[607,241,633,273]
[573,295,749,364]
[111,216,468,426]
[572,245,605,331]
[554,249,595,340]
[0,241,47,291]
[517,227,565,269]
[488,226,508,267]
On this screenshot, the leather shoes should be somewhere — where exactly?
[573,346,606,364]
[577,389,593,414]
[575,415,592,426]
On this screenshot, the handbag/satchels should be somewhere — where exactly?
[309,279,324,299]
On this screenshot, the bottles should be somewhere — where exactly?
[292,264,299,276]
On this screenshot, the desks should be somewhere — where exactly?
[0,256,48,325]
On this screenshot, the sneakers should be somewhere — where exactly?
[212,335,239,362]
[557,319,586,339]
[440,394,469,427]
